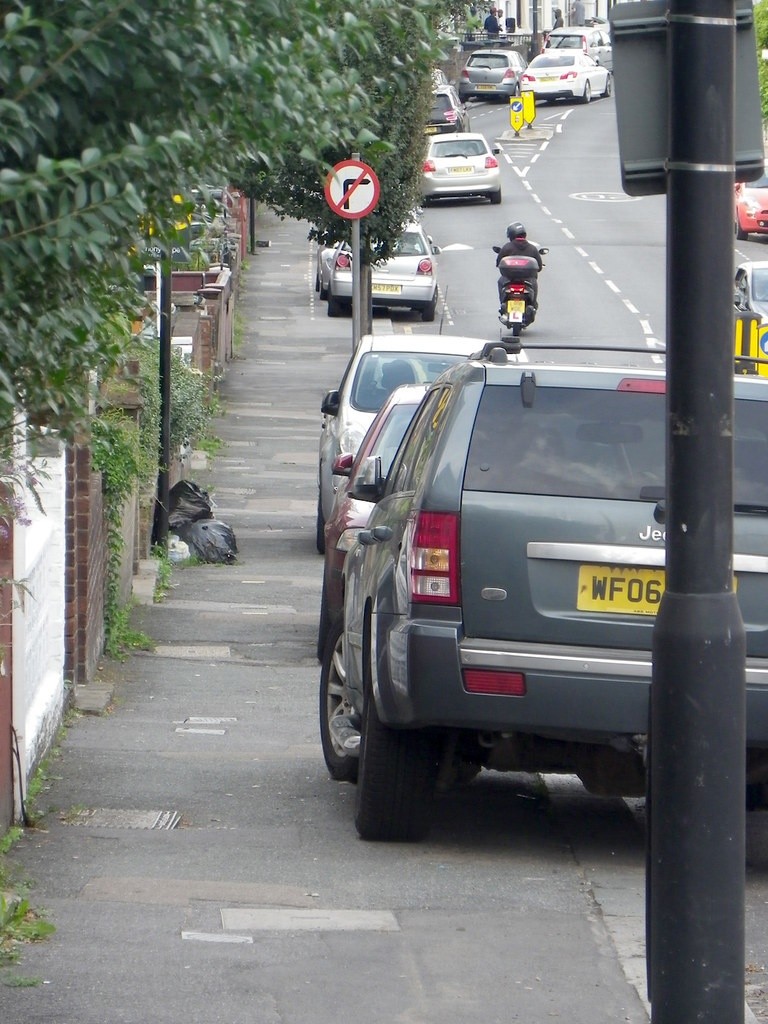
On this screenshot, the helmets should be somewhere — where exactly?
[507,222,527,241]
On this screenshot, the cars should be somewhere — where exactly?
[316,335,529,669]
[459,49,529,104]
[320,337,767,843]
[540,26,613,71]
[315,207,441,321]
[734,261,768,329]
[518,53,611,103]
[421,68,470,133]
[734,171,768,240]
[417,133,501,205]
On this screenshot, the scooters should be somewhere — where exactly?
[493,245,549,337]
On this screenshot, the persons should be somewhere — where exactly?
[553,9,563,30]
[495,222,542,313]
[483,8,506,39]
